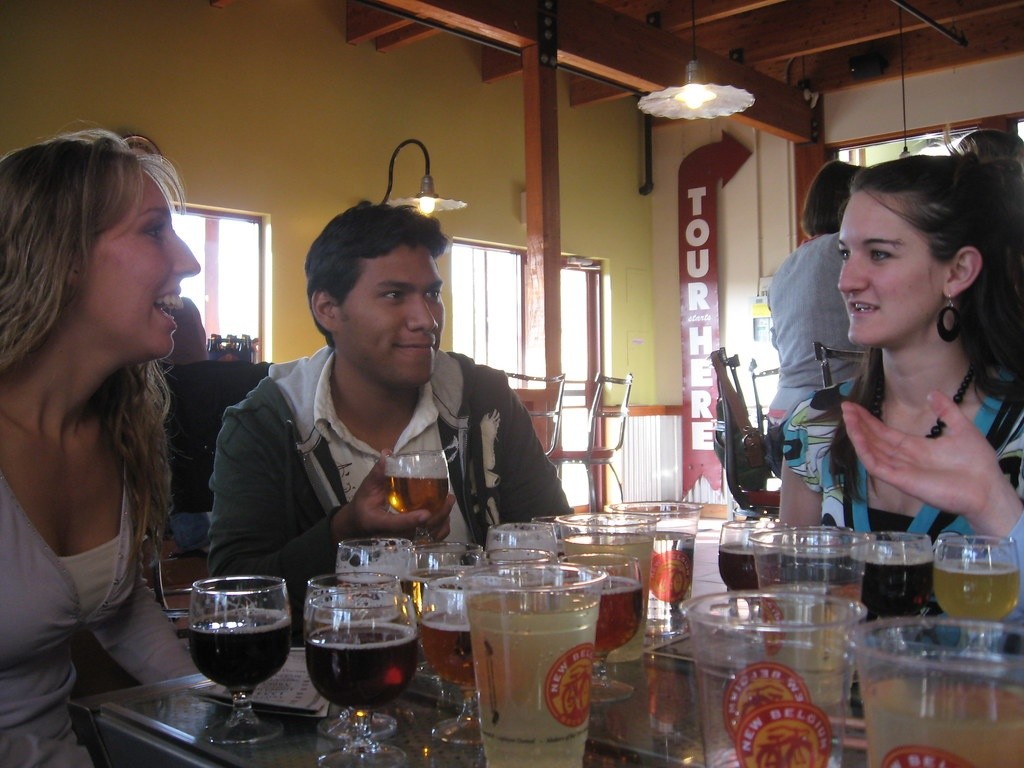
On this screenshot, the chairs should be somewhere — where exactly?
[709,346,780,519]
[506,372,633,514]
[150,362,275,623]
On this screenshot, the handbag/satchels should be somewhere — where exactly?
[714,403,770,491]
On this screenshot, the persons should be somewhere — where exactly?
[0,128,203,768]
[161,296,276,557]
[207,199,574,643]
[766,130,1024,653]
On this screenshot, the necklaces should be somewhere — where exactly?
[872,362,974,438]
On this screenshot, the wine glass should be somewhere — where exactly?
[408,542,482,680]
[934,535,1019,661]
[420,577,482,744]
[557,553,642,703]
[708,521,794,644]
[387,450,450,554]
[302,572,418,768]
[187,575,291,743]
[862,529,935,653]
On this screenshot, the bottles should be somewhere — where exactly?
[207,334,253,363]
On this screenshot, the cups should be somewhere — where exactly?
[531,517,569,557]
[747,526,870,706]
[555,512,659,661]
[605,500,703,636]
[464,561,608,768]
[486,522,557,561]
[848,616,1024,768]
[336,538,422,622]
[478,549,557,565]
[676,592,868,768]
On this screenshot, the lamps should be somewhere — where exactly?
[636,0,756,119]
[379,139,468,214]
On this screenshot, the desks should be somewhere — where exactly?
[65,581,1024,768]
